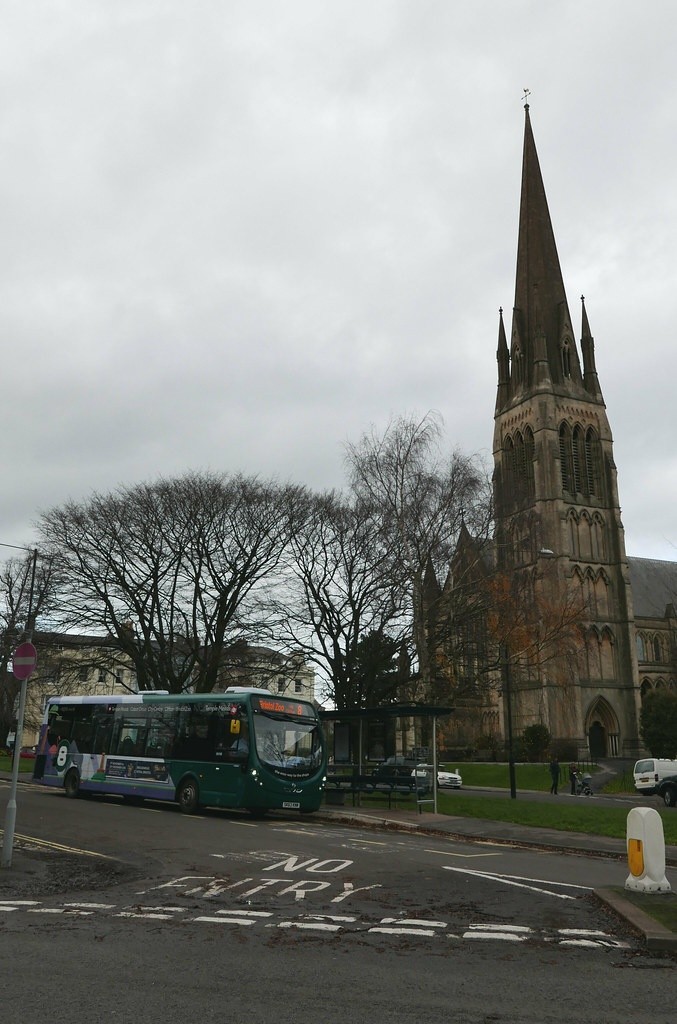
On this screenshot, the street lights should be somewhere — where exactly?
[503,548,555,797]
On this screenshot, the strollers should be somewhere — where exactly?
[573,771,594,796]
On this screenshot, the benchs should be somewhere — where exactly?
[325,761,436,814]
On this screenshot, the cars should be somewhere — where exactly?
[411,763,463,789]
[656,774,677,807]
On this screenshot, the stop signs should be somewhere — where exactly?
[12,642,37,680]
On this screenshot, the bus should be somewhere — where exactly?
[32,686,329,818]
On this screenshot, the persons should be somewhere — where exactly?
[568,761,577,796]
[547,756,562,797]
[228,729,251,764]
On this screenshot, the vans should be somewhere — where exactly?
[633,758,677,796]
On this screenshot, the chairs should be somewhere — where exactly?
[49,719,206,760]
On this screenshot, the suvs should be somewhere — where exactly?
[361,764,414,795]
